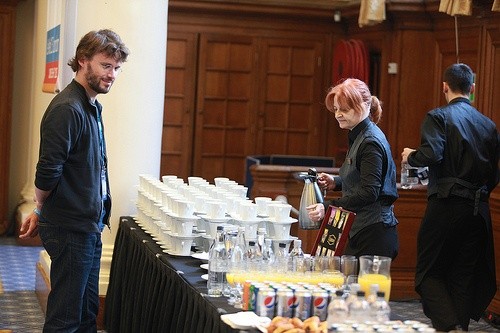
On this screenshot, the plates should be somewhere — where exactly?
[200,264,209,271]
[191,252,210,263]
[201,273,209,281]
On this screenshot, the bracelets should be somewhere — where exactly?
[34,208,43,216]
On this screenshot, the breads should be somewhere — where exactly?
[267,315,330,333]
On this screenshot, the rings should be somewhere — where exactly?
[319,217,321,219]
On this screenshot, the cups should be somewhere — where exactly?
[133,174,297,257]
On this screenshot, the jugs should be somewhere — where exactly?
[298,168,327,230]
[359,256,392,303]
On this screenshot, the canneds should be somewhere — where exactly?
[327,319,437,333]
[242,280,338,321]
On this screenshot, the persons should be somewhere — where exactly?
[20,29,130,333]
[304,78,399,274]
[401,62,500,333]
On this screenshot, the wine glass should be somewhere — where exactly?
[225,254,358,309]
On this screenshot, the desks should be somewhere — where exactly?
[103,217,441,333]
[250,162,499,318]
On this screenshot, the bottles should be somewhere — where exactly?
[367,283,379,302]
[207,226,304,297]
[417,164,431,186]
[327,290,348,320]
[371,291,390,323]
[350,292,371,322]
[407,160,419,185]
[400,161,411,190]
